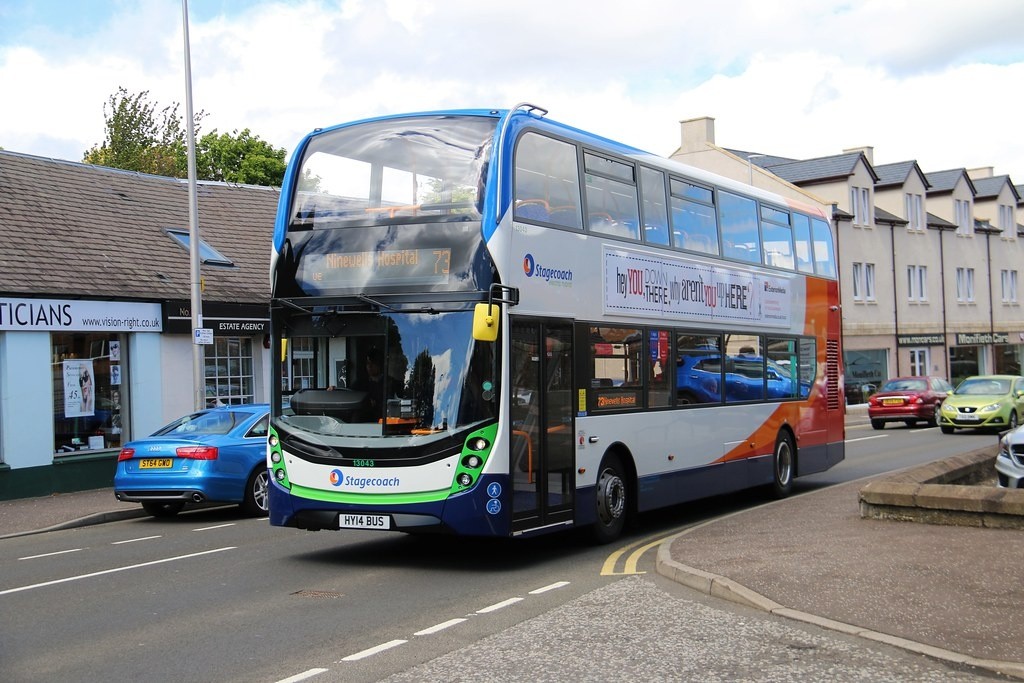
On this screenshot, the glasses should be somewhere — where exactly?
[79,371,88,387]
[111,344,119,350]
[114,374,119,376]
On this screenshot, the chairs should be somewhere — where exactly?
[613,220,637,239]
[549,205,577,227]
[723,240,733,257]
[644,224,666,243]
[516,199,550,222]
[673,229,688,248]
[689,233,711,252]
[588,212,613,225]
[750,246,785,265]
[734,243,749,260]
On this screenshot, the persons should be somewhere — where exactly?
[111,390,121,409]
[78,361,94,413]
[109,341,120,360]
[111,366,121,385]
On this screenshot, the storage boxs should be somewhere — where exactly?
[88,436,105,449]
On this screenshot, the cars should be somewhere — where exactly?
[993,422,1024,490]
[936,374,1024,436]
[114,401,294,518]
[666,349,810,406]
[866,376,957,430]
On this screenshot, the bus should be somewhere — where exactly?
[264,102,847,549]
